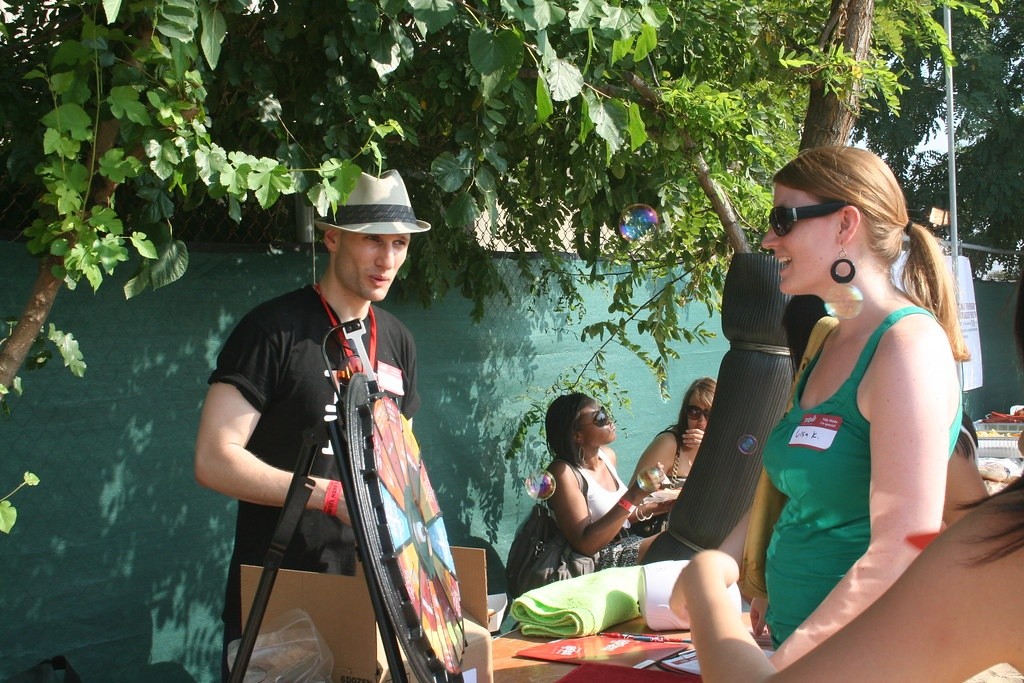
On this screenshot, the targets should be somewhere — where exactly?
[361,393,469,678]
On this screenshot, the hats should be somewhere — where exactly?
[314,168,432,234]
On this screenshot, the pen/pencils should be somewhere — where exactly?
[598,632,664,643]
[628,633,691,643]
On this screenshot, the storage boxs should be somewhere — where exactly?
[228,540,497,683]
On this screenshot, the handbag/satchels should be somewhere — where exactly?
[503,460,596,601]
[629,430,682,536]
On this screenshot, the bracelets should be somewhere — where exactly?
[322,477,343,515]
[617,497,639,515]
[636,501,654,521]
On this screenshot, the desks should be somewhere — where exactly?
[492,610,771,683]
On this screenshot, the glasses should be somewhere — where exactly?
[684,405,711,422]
[337,354,362,387]
[769,201,853,238]
[580,406,609,428]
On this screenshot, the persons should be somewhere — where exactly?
[671,278,1024,683]
[781,292,988,531]
[193,168,433,683]
[627,377,717,536]
[746,145,971,670]
[544,393,668,573]
